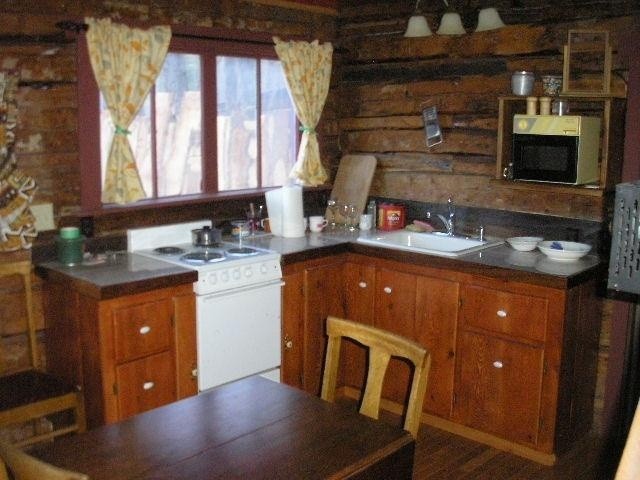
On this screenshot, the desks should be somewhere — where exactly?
[23,374,416,480]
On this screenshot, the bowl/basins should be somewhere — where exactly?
[505,236,543,251]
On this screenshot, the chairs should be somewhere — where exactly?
[0,260,88,452]
[321,316,432,439]
[1,436,90,480]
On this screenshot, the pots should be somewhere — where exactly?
[190,225,218,246]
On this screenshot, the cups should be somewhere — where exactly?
[308,215,328,232]
[359,214,374,230]
[261,218,272,233]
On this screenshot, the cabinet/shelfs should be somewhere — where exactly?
[342,262,414,408]
[455,282,603,456]
[41,293,198,427]
[415,275,458,421]
[282,264,343,396]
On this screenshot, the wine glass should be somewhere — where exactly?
[327,199,342,229]
[340,204,350,229]
[348,205,359,232]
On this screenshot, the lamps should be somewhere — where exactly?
[404,1,505,38]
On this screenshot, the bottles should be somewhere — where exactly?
[57,227,85,266]
[367,201,377,227]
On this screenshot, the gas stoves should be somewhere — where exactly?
[133,238,272,267]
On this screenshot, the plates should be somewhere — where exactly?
[535,240,592,261]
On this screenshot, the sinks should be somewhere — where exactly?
[357,231,506,257]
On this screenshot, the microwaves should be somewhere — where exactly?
[508,113,600,186]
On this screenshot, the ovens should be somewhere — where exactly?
[191,257,282,391]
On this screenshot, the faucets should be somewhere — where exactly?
[427,197,456,235]
